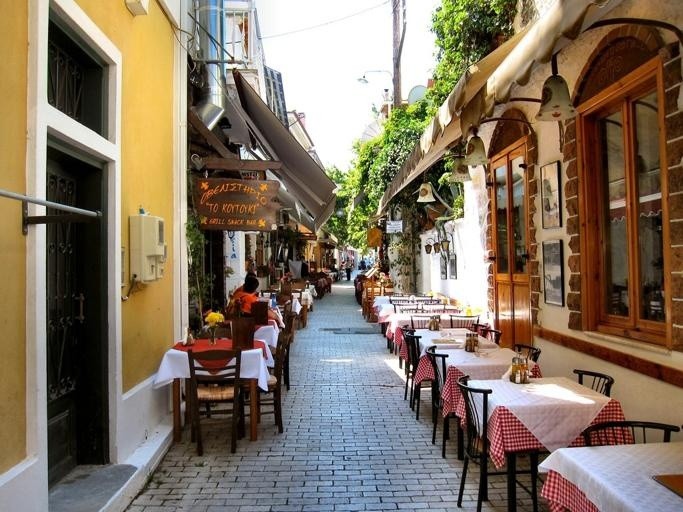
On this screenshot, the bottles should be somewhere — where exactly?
[511,356,531,384]
[187,328,194,346]
[305,279,308,289]
[428,314,440,330]
[465,333,478,352]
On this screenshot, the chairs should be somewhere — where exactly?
[362,280,683,512]
[153,276,333,456]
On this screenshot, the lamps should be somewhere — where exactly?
[417,97,543,212]
[535,17,683,121]
[425,232,454,254]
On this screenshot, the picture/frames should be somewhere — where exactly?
[542,239,564,307]
[540,159,562,229]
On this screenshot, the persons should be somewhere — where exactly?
[280,270,289,281]
[322,261,352,281]
[360,259,366,270]
[228,272,278,319]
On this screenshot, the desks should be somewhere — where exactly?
[537,439,682,512]
[378,304,461,338]
[399,327,496,420]
[455,377,635,512]
[372,296,450,334]
[385,313,492,356]
[435,347,543,461]
